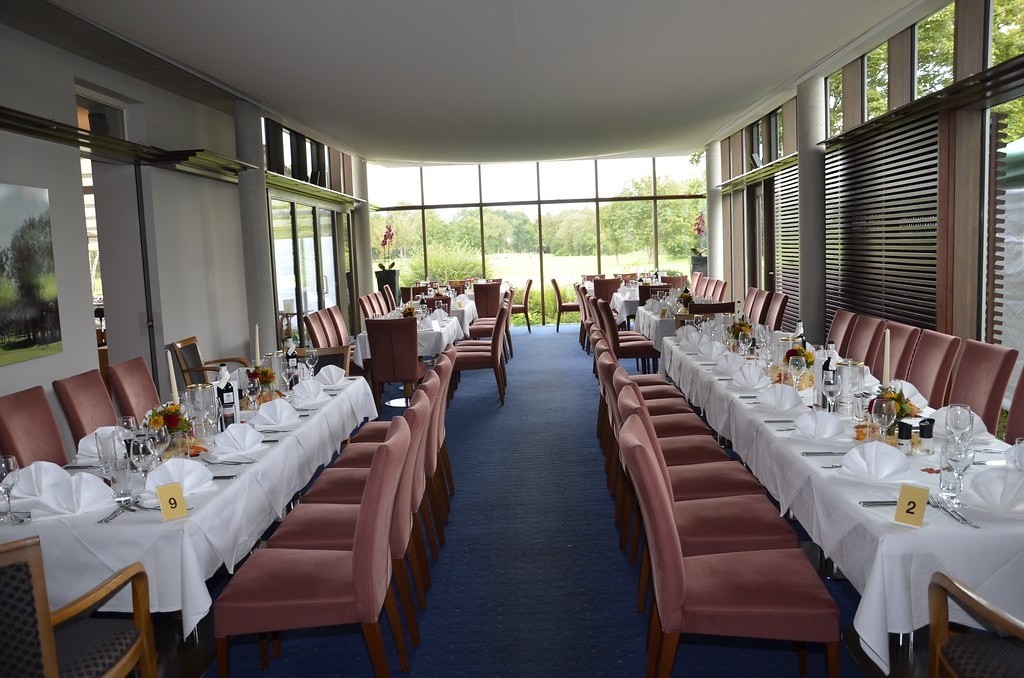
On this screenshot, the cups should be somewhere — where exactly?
[170,432,189,461]
[186,383,219,447]
[1016,438,1024,471]
[95,430,118,474]
[109,453,131,504]
[435,300,448,313]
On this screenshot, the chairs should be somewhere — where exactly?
[551,271,843,678]
[827,309,1024,444]
[303,279,533,406]
[213,343,457,678]
[1,343,161,677]
[173,336,250,386]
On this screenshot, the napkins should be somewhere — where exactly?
[2,364,347,522]
[675,325,1024,521]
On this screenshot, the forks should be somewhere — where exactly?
[927,493,981,529]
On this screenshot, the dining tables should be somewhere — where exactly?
[662,336,1024,675]
[353,316,465,370]
[610,293,639,326]
[634,306,675,352]
[0,375,378,642]
[399,290,479,338]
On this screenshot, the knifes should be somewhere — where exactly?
[97,495,140,524]
[201,458,255,466]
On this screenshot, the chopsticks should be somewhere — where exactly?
[699,363,716,366]
[763,420,794,424]
[858,501,898,507]
[717,378,733,382]
[213,475,236,480]
[739,395,756,399]
[801,451,846,456]
[300,414,308,417]
[262,440,278,442]
[687,353,699,356]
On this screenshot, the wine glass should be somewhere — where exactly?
[650,287,715,318]
[241,349,325,420]
[117,416,170,502]
[465,282,470,291]
[0,455,32,525]
[692,312,975,510]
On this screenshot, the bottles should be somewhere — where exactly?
[286,336,299,390]
[420,292,427,311]
[822,339,835,409]
[791,318,807,356]
[216,363,235,433]
[683,288,690,308]
[445,282,450,293]
[736,301,745,322]
